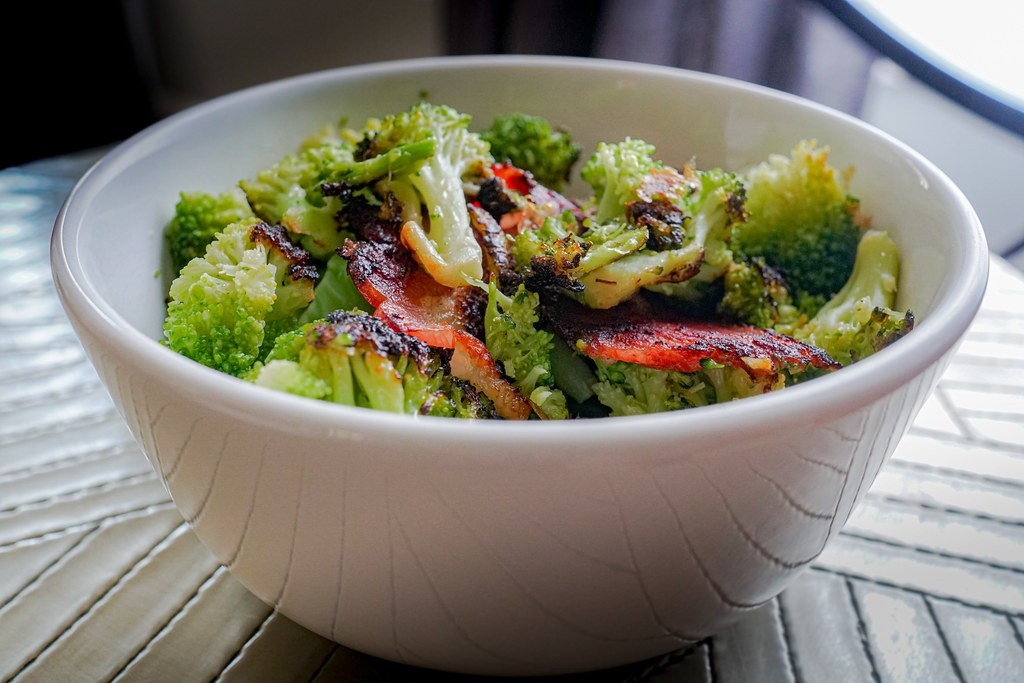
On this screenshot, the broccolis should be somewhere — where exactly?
[161,101,912,423]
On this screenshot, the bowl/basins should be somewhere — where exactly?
[50,55,990,679]
